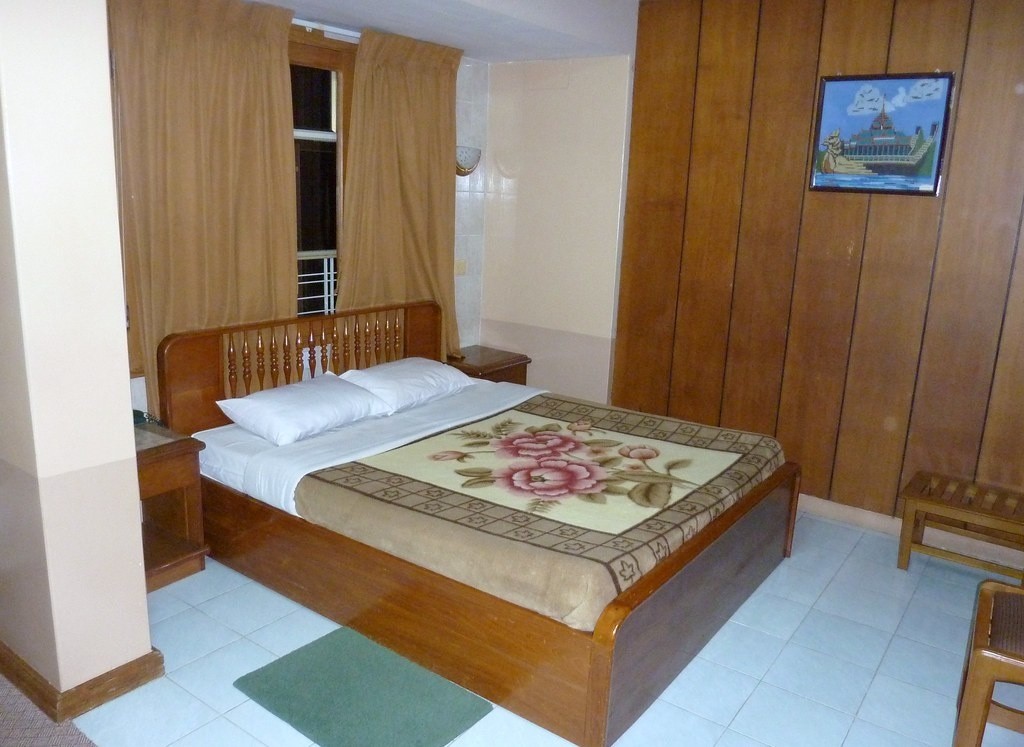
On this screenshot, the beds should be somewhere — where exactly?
[156,300,802,747]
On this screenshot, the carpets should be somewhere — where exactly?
[233,627,493,747]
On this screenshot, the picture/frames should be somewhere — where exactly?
[808,71,954,197]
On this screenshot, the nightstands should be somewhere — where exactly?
[447,344,533,387]
[134,421,209,594]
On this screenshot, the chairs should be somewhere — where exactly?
[952,579,1024,747]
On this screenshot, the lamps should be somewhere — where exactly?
[456,146,482,176]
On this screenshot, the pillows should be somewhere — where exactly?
[215,370,394,447]
[338,356,477,416]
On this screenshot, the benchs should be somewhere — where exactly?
[896,469,1024,586]
